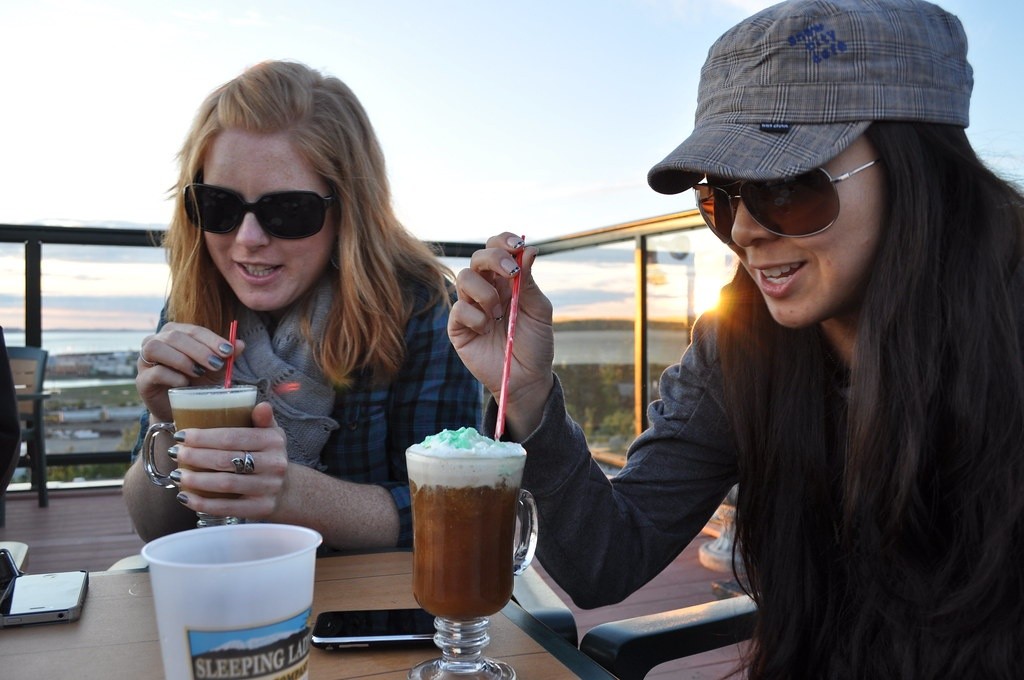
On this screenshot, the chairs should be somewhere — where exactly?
[0,346,49,528]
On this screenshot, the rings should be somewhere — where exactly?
[231,451,255,475]
[139,348,157,365]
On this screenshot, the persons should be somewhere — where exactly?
[105,60,494,581]
[445,0,1024,680]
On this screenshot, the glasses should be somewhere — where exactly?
[184,168,339,240]
[693,158,881,244]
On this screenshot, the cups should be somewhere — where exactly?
[143,523,323,680]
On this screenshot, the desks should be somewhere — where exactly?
[0,550,620,680]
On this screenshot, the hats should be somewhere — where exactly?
[648,0,974,195]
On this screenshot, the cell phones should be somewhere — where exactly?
[0,569,89,626]
[311,608,440,651]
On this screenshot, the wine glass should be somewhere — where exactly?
[143,386,259,529]
[406,447,538,680]
